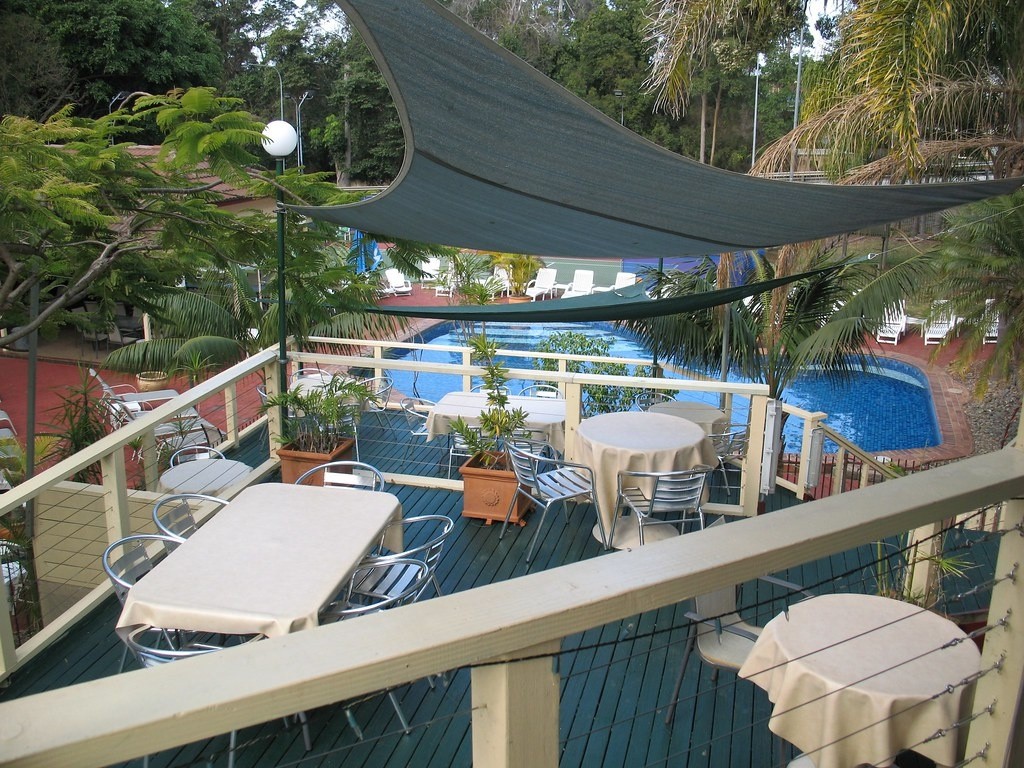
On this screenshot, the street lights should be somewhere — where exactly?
[751,52,767,170]
[241,61,284,175]
[260,119,300,448]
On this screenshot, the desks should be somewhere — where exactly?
[112,481,404,753]
[738,593,984,768]
[648,400,728,456]
[96,315,143,338]
[424,391,569,463]
[563,414,720,550]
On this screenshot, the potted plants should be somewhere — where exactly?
[260,376,381,485]
[458,334,537,527]
[863,463,975,629]
[0,430,62,540]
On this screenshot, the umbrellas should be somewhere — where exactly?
[347,228,383,275]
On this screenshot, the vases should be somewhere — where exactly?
[137,371,168,392]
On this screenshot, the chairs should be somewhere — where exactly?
[981,298,1001,344]
[665,514,765,728]
[870,299,908,345]
[174,219,860,333]
[71,300,145,359]
[8,326,29,351]
[0,365,755,768]
[920,301,962,346]
